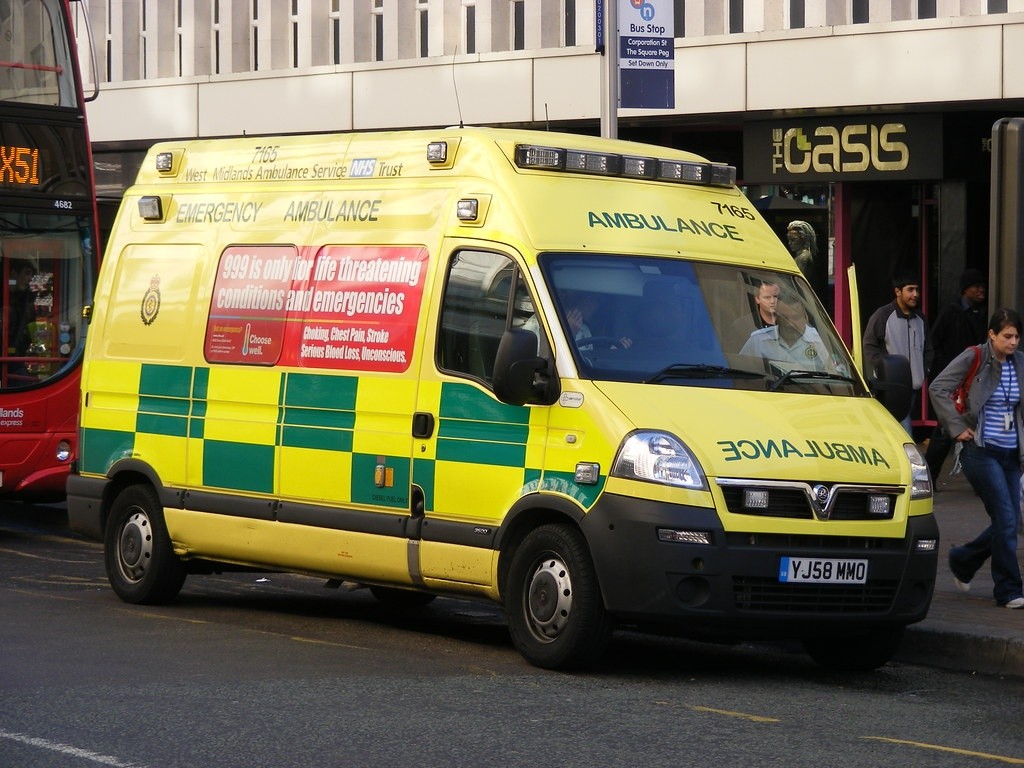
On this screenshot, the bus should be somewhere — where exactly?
[0,0,105,495]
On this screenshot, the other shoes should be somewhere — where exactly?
[955,576,970,593]
[932,480,936,490]
[1005,598,1024,608]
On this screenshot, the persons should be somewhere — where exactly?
[0,259,35,372]
[524,287,632,358]
[926,269,988,491]
[928,309,1024,610]
[787,220,820,282]
[863,276,924,438]
[721,276,838,378]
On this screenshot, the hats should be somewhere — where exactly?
[959,268,988,294]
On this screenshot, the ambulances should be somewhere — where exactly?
[65,120,942,674]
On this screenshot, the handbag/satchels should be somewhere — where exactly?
[950,346,982,415]
